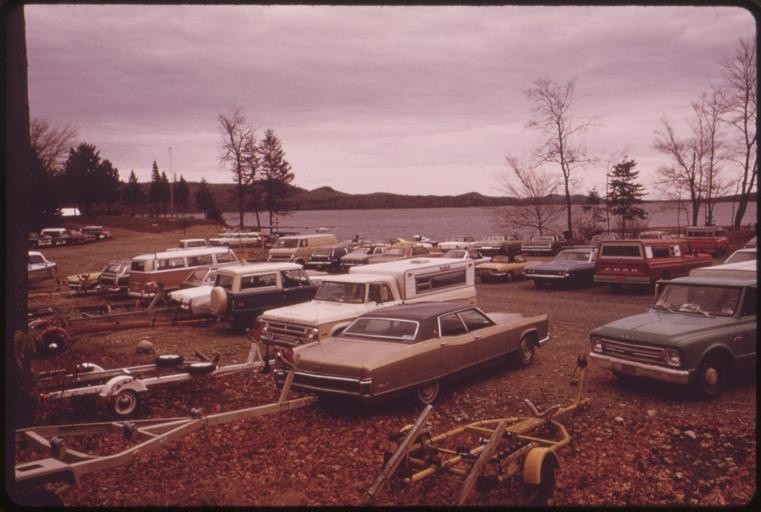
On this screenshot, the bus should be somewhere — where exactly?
[128,246,244,306]
[209,232,262,248]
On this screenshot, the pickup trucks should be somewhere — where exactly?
[590,260,757,399]
[521,236,559,255]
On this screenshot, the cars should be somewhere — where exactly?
[436,234,477,250]
[382,238,416,249]
[272,300,550,409]
[522,248,599,287]
[28,252,58,284]
[590,234,619,245]
[53,231,96,245]
[724,249,759,268]
[473,231,522,256]
[64,270,102,294]
[168,281,216,317]
[476,251,543,281]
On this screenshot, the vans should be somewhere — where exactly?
[40,228,68,244]
[267,230,301,244]
[179,239,208,249]
[638,231,672,243]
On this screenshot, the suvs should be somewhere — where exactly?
[306,247,353,271]
[98,260,132,298]
[684,227,729,254]
[78,227,112,240]
[594,239,714,291]
[339,244,388,271]
[368,244,428,264]
[211,261,321,327]
[181,267,231,288]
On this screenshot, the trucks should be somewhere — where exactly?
[254,258,478,376]
[266,235,338,271]
